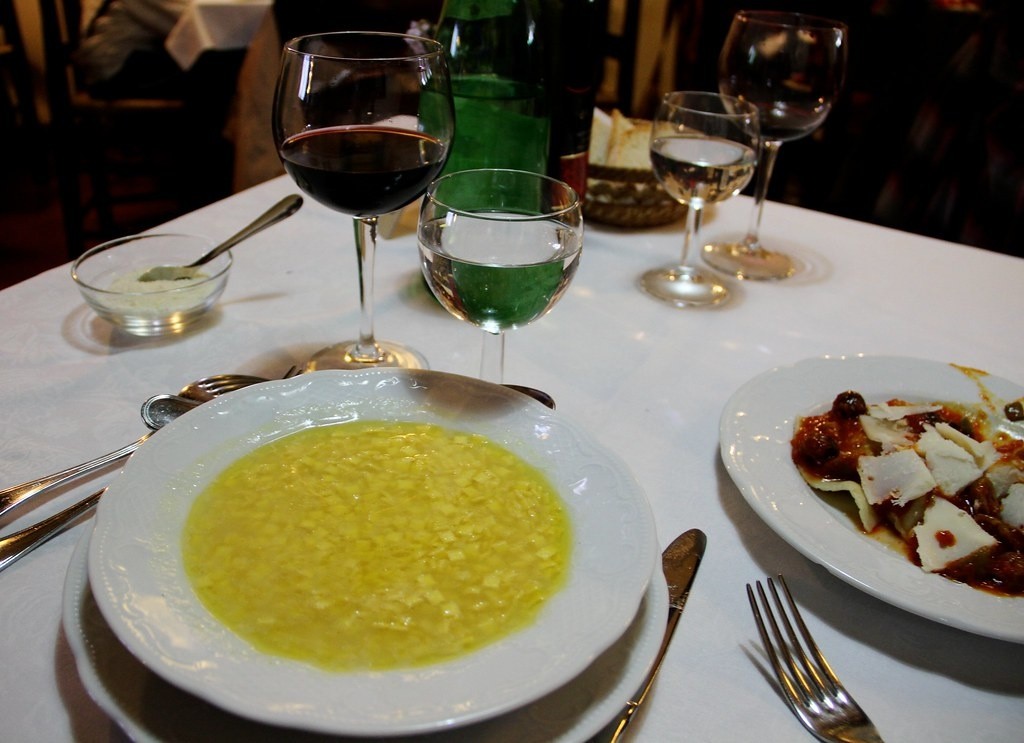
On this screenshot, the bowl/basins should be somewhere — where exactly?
[588,528,708,743]
[71,232,234,337]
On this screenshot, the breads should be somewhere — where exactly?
[602,109,681,170]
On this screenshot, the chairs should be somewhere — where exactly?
[41,0,183,258]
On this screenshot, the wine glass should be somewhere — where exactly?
[418,168,585,412]
[269,31,457,372]
[638,90,764,310]
[700,10,847,280]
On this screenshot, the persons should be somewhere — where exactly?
[79,0,241,209]
[229,0,609,195]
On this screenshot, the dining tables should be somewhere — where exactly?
[0,114,1024,743]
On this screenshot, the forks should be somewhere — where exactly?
[745,573,884,743]
[0,368,305,572]
[0,374,270,519]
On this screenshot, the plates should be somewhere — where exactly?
[719,352,1024,644]
[59,519,671,743]
[86,366,658,739]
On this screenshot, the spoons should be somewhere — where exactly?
[137,193,304,282]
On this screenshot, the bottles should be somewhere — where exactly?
[418,0,556,306]
